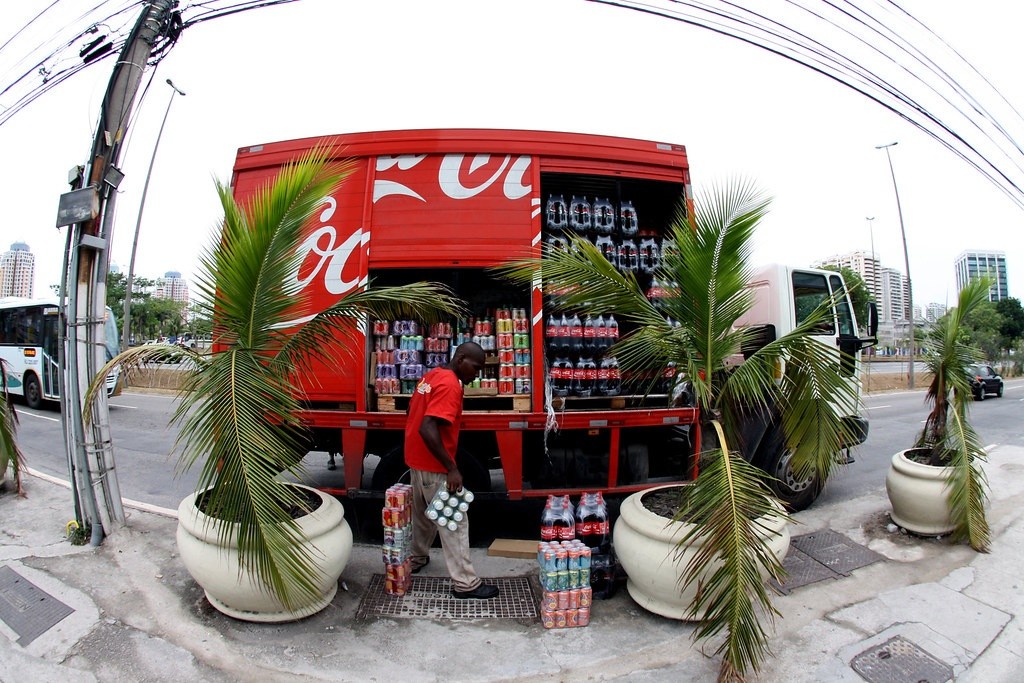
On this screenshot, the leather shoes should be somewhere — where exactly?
[411,556,430,574]
[453,584,499,599]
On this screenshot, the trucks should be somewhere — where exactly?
[211,128,879,519]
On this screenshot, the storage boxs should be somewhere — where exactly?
[487,539,540,559]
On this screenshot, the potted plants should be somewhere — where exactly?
[484,174,872,683]
[885,277,1001,553]
[76,132,476,622]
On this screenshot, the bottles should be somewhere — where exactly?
[540,491,618,598]
[540,193,697,409]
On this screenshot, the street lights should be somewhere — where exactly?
[120,77,188,388]
[876,141,917,390]
[863,216,877,309]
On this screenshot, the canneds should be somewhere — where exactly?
[372,308,532,396]
[537,540,593,629]
[424,480,475,532]
[381,483,413,595]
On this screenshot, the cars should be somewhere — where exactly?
[126,335,199,370]
[965,361,1005,400]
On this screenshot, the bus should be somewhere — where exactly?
[0,296,123,411]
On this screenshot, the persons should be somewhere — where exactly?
[405,341,499,599]
[327,452,337,470]
